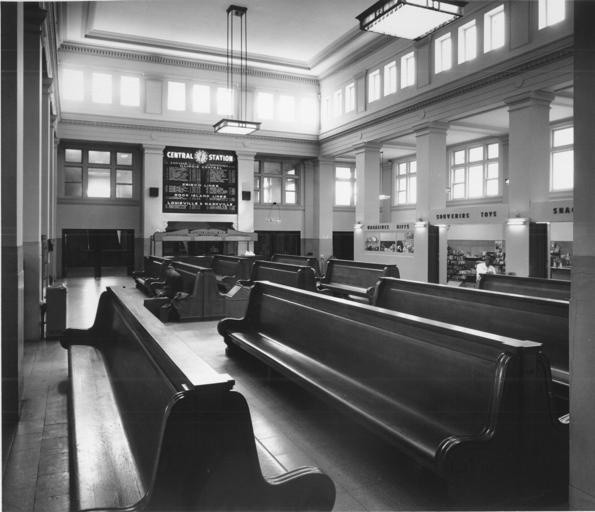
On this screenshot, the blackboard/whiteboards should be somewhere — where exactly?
[162,145,239,214]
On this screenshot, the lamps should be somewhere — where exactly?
[352,1,468,44]
[211,4,264,139]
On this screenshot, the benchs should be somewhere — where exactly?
[55,251,570,511]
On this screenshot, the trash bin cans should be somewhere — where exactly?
[46,286,67,341]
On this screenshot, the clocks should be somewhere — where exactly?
[193,149,209,165]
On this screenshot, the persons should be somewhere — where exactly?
[307,253,313,262]
[475,256,497,286]
[209,245,217,254]
